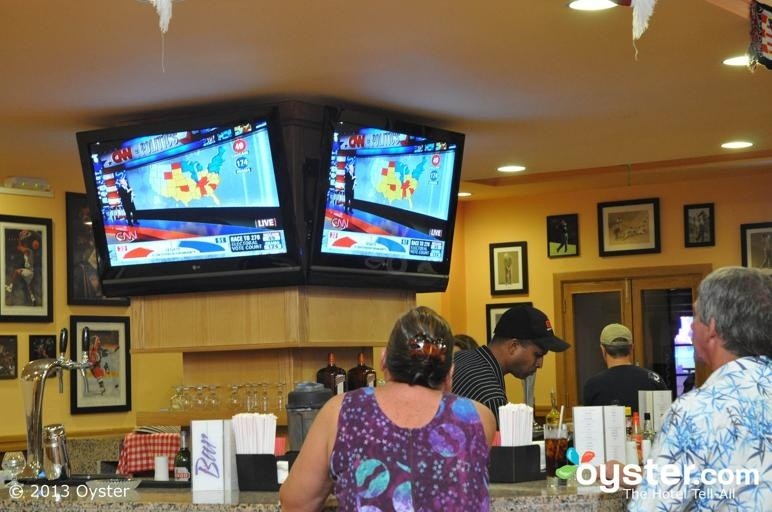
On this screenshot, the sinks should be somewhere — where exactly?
[67,472,130,488]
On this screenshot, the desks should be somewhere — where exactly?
[114,430,287,475]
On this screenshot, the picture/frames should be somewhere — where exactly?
[597,197,661,256]
[740,221,772,268]
[684,201,715,247]
[547,212,579,258]
[485,303,531,346]
[28,334,56,379]
[66,191,130,306]
[70,315,131,414]
[0,335,18,379]
[490,241,528,294]
[1,215,52,322]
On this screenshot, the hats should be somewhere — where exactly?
[495,305,572,354]
[599,322,635,347]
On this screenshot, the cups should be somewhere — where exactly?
[543,422,568,477]
[152,456,171,481]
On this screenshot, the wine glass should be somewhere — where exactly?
[1,451,26,488]
[168,382,288,414]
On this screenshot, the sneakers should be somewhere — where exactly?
[31,296,37,306]
[5,284,13,294]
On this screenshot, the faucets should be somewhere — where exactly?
[17,326,98,478]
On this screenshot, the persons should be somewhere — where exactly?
[759,233,772,268]
[5,229,39,306]
[278,306,497,512]
[557,219,568,252]
[583,323,669,417]
[344,165,356,215]
[503,253,513,284]
[627,265,772,512]
[450,304,572,432]
[452,334,479,360]
[695,210,707,243]
[89,335,119,395]
[118,178,140,226]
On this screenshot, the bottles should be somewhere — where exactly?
[174,431,192,483]
[625,406,655,466]
[347,352,377,392]
[42,424,72,479]
[546,399,561,428]
[317,351,348,396]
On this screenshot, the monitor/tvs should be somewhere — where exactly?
[76,115,308,299]
[310,105,466,293]
